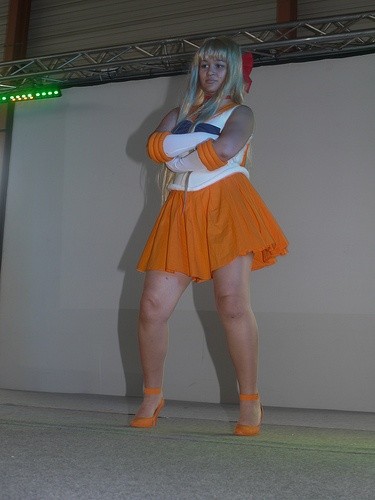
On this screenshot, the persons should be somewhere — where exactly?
[127,36,291,437]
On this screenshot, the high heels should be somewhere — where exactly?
[233,388,263,435]
[130,384,167,428]
